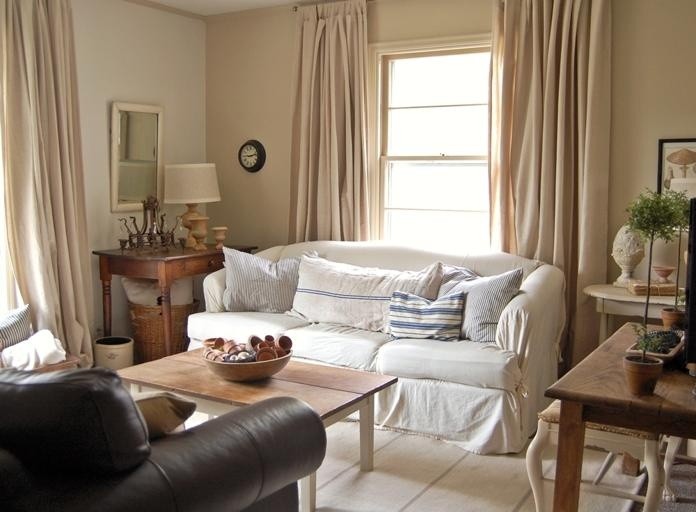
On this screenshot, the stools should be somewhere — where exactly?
[524,398,683,511]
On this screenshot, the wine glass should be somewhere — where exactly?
[210,226,229,251]
[188,217,209,250]
[653,266,674,283]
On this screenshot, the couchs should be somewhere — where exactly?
[186,240,567,455]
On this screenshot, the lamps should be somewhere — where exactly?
[163,163,222,247]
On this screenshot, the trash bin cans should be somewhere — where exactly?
[93,336,134,370]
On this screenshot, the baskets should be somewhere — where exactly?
[128,299,200,364]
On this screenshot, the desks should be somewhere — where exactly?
[544,321,696,511]
[92,245,259,355]
[583,284,686,347]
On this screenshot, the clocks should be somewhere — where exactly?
[237,139,266,174]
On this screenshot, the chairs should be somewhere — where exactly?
[33,353,80,371]
[0,366,327,512]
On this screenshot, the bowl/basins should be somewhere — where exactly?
[202,351,293,383]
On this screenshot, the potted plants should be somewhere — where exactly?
[623,188,676,397]
[626,329,686,359]
[661,188,692,331]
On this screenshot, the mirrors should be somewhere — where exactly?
[110,101,163,213]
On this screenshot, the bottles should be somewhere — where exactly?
[610,224,647,288]
[170,215,189,248]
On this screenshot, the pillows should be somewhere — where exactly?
[135,390,197,441]
[119,272,196,307]
[0,302,31,350]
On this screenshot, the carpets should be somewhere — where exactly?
[117,412,648,512]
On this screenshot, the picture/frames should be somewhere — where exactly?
[657,138,696,200]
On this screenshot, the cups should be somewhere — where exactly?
[202,335,292,362]
